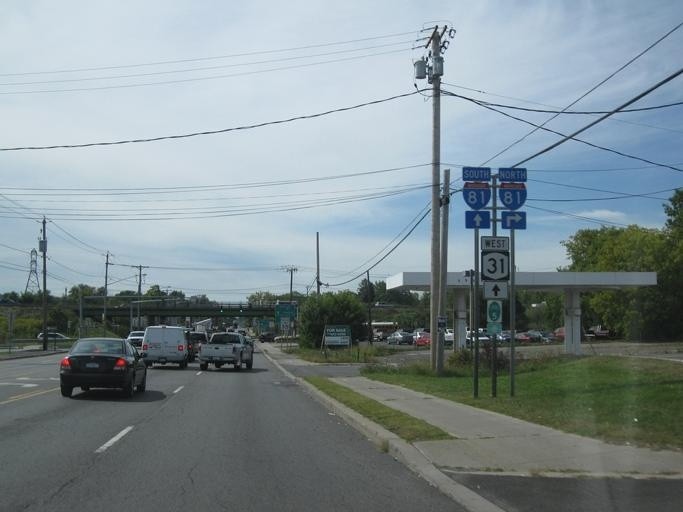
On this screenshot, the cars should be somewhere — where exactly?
[60,324,275,398]
[373,327,566,346]
[38,333,69,340]
[584,330,595,337]
